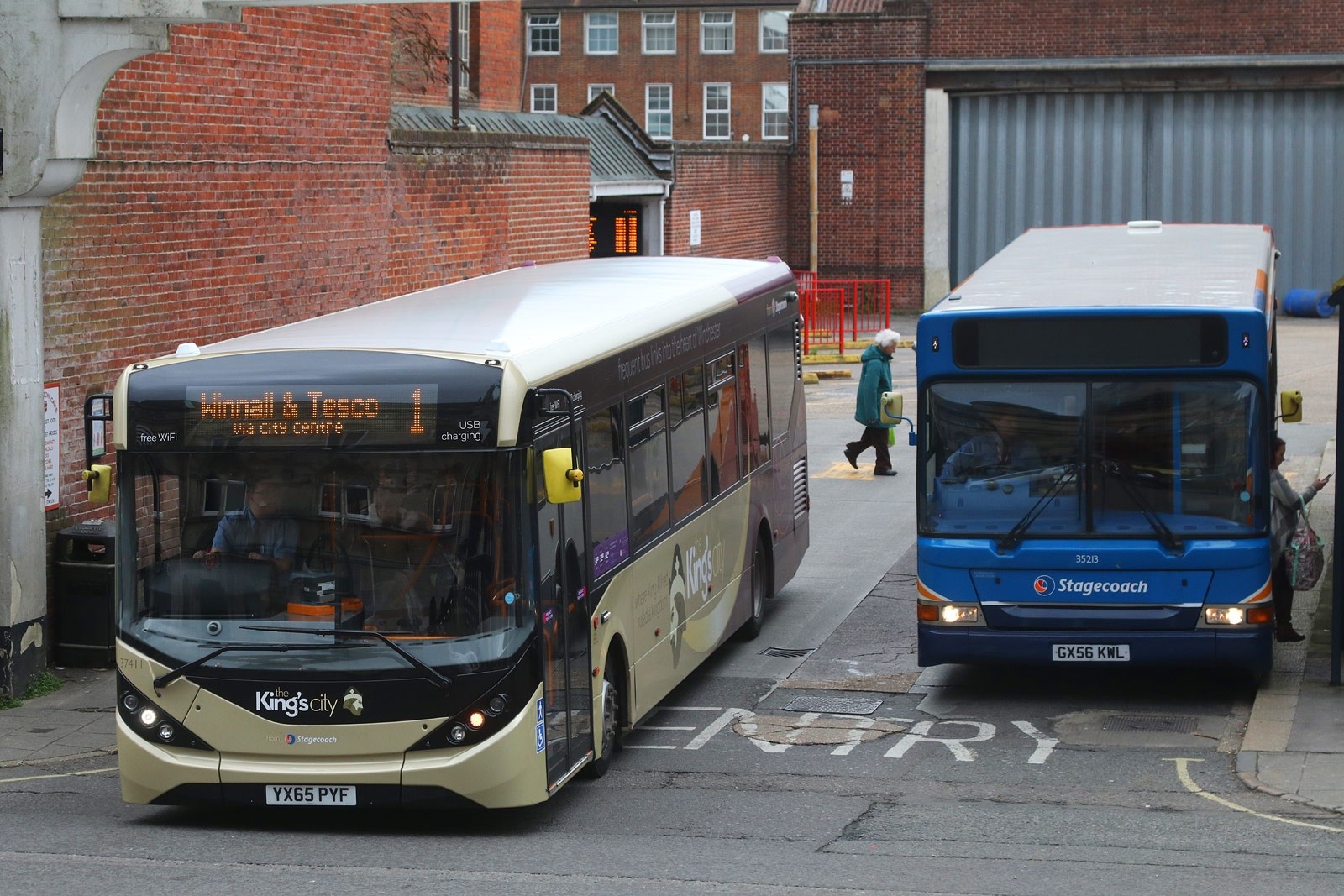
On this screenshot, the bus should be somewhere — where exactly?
[880,220,1302,676]
[82,256,811,812]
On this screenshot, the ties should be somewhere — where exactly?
[243,522,263,557]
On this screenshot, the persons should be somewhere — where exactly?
[210,475,300,573]
[844,330,898,476]
[368,485,422,533]
[941,407,1037,481]
[1269,436,1329,642]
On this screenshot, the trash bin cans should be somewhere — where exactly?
[52,520,117,668]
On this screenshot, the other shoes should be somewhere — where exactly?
[844,449,858,470]
[874,469,898,476]
[1277,630,1306,642]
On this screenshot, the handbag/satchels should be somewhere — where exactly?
[887,427,895,447]
[1284,495,1325,591]
